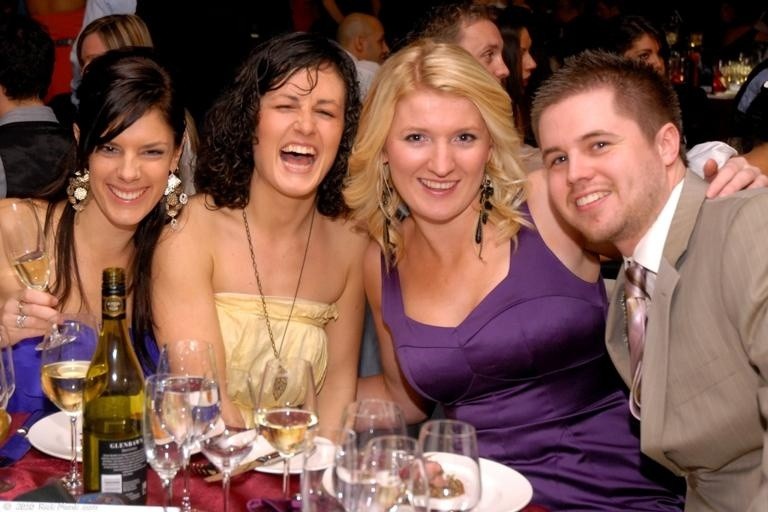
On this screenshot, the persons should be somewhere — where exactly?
[528,50,768,512]
[335,0,768,162]
[1,1,200,204]
[344,39,768,512]
[1,44,188,465]
[147,30,368,451]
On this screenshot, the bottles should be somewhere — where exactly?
[662,32,704,87]
[82,266,148,505]
[712,59,727,93]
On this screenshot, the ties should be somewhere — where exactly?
[623,264,647,422]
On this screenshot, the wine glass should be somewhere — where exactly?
[1,324,17,496]
[39,312,319,511]
[0,198,78,352]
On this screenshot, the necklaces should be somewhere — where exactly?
[241,206,315,403]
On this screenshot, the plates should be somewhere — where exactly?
[320,450,535,511]
[198,429,339,474]
[29,409,203,463]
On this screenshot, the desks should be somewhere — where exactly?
[0,411,549,512]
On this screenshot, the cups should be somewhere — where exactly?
[300,398,482,511]
